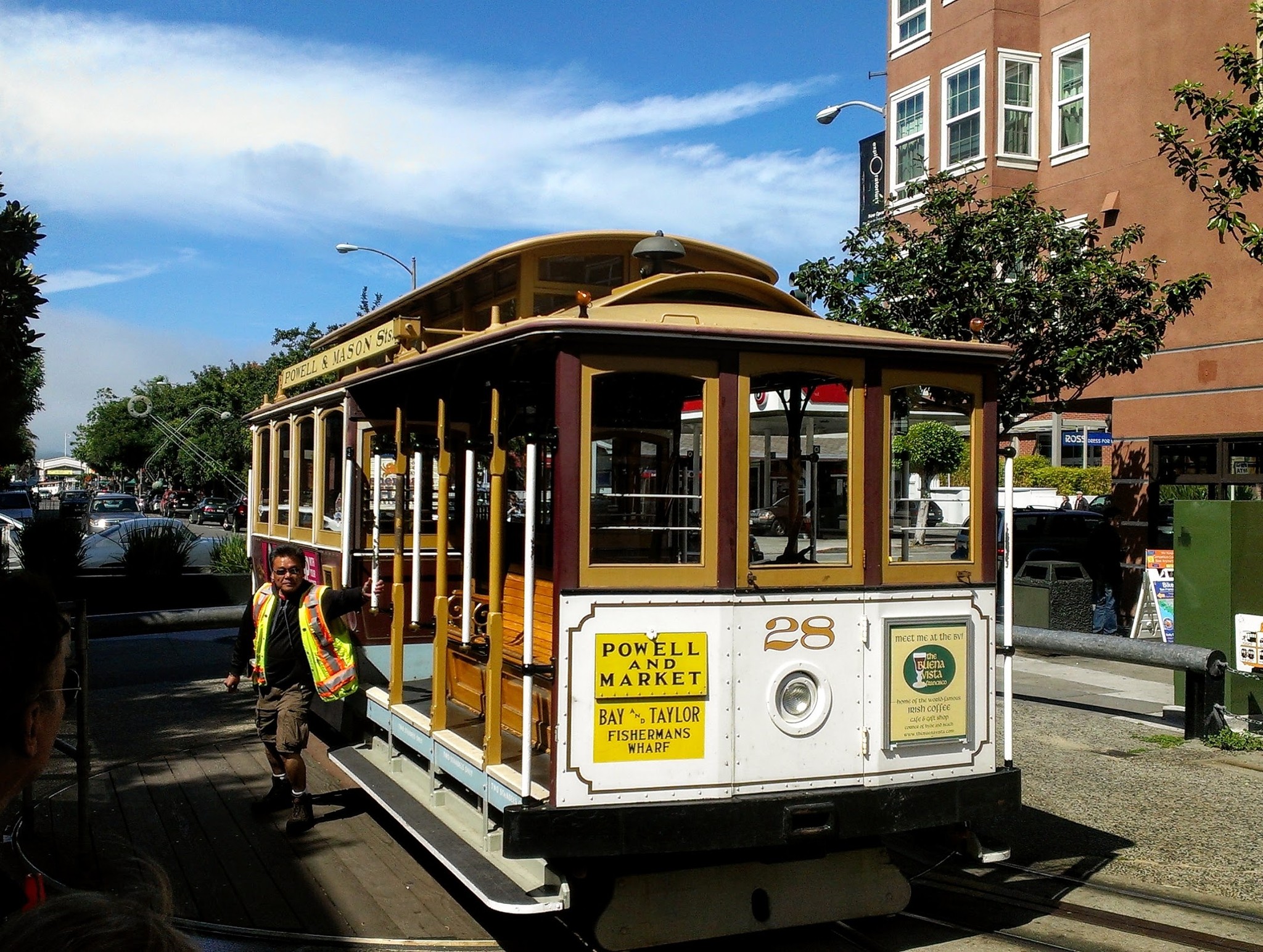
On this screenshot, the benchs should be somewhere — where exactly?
[447,574,555,747]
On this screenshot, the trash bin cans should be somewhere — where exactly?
[1013,560,1096,654]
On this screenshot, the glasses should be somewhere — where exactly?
[273,567,302,576]
[31,668,82,708]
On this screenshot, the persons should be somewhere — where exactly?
[507,493,522,522]
[1089,505,1125,638]
[1060,490,1089,512]
[224,544,385,836]
[0,573,205,952]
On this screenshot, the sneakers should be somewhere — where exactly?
[248,775,293,815]
[285,790,315,836]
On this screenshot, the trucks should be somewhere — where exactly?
[31,481,64,496]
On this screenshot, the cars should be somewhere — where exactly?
[0,491,33,525]
[0,513,26,571]
[189,497,232,525]
[38,490,146,544]
[77,518,223,569]
[138,490,200,518]
[221,491,249,533]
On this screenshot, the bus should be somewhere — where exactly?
[240,230,1022,952]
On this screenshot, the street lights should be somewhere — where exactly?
[336,241,418,291]
[54,475,59,481]
[46,475,51,481]
